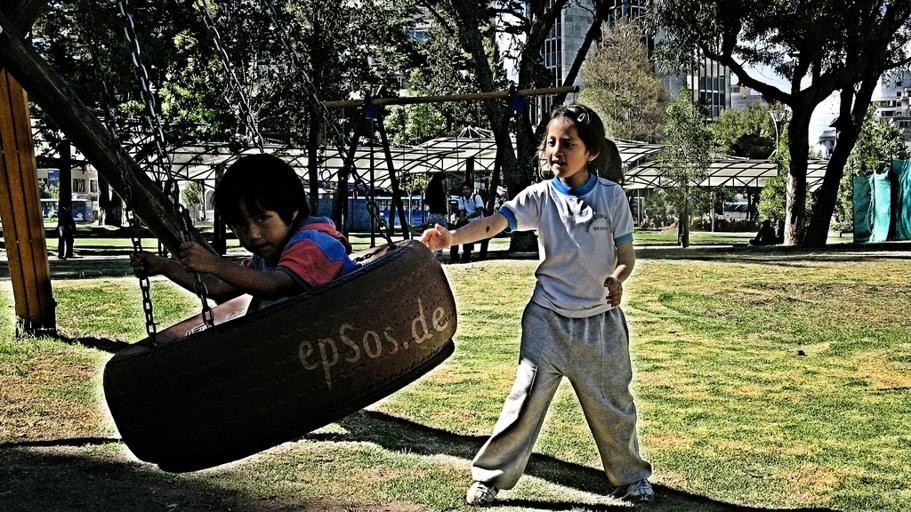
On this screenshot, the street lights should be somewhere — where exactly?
[767,110,789,177]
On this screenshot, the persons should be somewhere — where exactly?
[448,181,484,263]
[131,154,358,314]
[749,220,784,245]
[57,201,75,257]
[426,172,448,262]
[418,105,655,505]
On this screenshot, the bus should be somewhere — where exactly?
[374,195,465,230]
[40,199,93,221]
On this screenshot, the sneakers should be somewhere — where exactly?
[448,251,472,264]
[628,479,655,504]
[466,481,500,505]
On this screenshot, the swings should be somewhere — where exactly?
[401,97,482,236]
[72,2,456,472]
